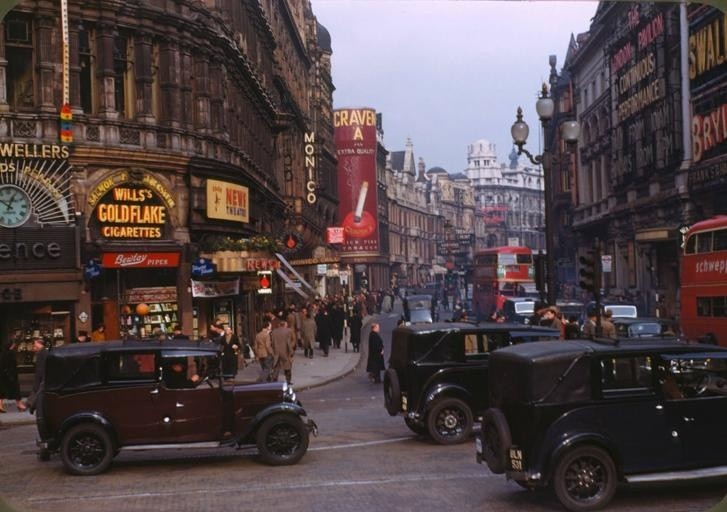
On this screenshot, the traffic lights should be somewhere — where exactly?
[578,254,602,289]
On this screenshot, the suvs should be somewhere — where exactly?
[383,320,563,448]
[472,335,727,510]
[24,339,319,479]
[396,285,440,324]
[504,293,664,340]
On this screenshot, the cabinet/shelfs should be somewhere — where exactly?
[119,303,180,334]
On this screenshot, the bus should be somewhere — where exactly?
[676,213,727,347]
[469,244,535,319]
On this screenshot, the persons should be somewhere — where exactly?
[487,309,615,340]
[20,325,106,411]
[366,324,385,383]
[432,284,481,323]
[255,311,297,379]
[286,292,394,359]
[210,318,239,380]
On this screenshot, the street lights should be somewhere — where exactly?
[444,219,456,270]
[506,79,587,305]
